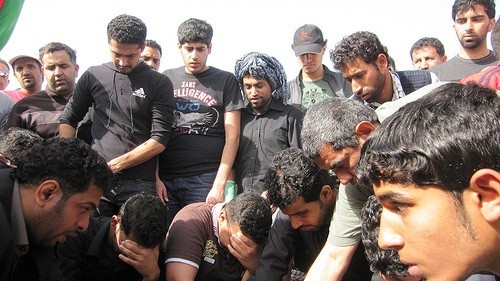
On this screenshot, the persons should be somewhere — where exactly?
[0,0,500,281]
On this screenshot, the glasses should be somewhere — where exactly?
[0,71,9,79]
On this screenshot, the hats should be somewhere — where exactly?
[8,50,43,67]
[292,24,324,57]
